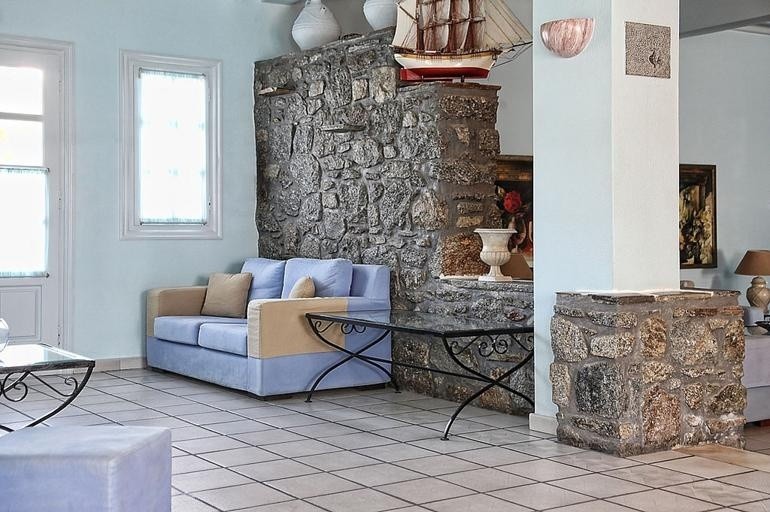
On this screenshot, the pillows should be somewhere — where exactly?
[200,272,315,318]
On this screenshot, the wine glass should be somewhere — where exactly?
[508,216,527,256]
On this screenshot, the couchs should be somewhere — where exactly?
[145,257,392,400]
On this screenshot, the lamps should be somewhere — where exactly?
[734,250,770,316]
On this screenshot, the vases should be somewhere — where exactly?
[473,228,519,281]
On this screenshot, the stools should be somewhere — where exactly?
[0,427,172,512]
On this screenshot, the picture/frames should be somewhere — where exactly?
[679,163,718,269]
[497,154,533,268]
